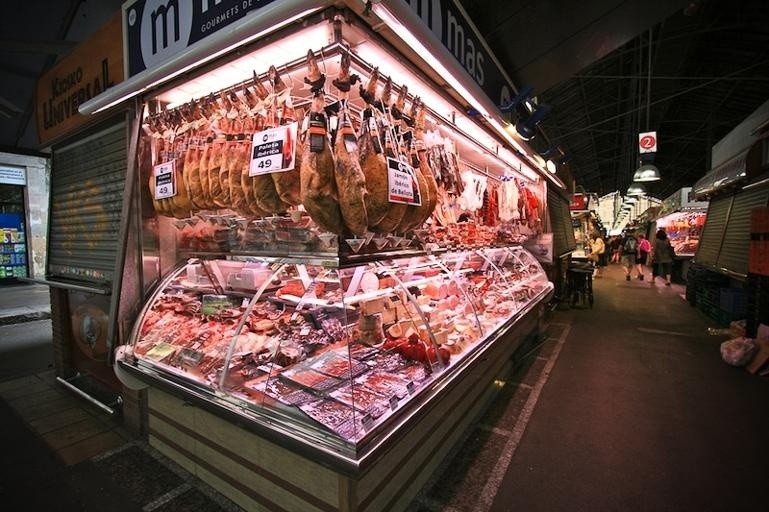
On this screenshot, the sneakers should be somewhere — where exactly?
[626,274,671,286]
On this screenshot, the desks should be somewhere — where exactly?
[565,269,593,308]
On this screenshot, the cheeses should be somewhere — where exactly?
[352,293,468,347]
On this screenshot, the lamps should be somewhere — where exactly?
[77,1,338,117]
[368,0,572,191]
[607,0,661,238]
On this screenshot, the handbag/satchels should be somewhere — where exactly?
[667,242,676,258]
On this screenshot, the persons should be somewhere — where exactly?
[618,229,641,281]
[647,230,675,286]
[634,233,648,280]
[583,232,624,269]
